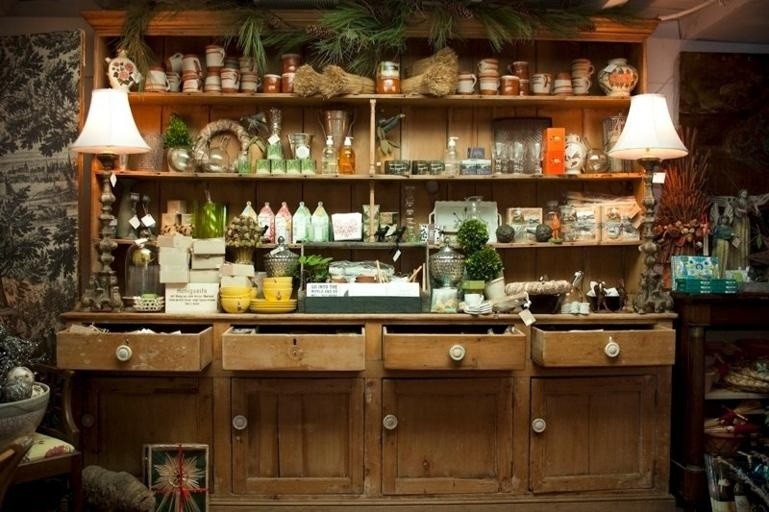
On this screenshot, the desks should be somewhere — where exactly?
[0,433,81,512]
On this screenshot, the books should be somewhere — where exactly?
[675,279,737,294]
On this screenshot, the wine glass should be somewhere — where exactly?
[492,140,542,176]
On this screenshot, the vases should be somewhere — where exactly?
[108,49,136,93]
[597,57,639,97]
[0,381,50,441]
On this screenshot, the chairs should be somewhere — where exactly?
[0,436,32,508]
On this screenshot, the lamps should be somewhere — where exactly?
[606,95,690,314]
[69,88,151,314]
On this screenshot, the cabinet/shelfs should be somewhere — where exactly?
[57,313,679,506]
[673,290,769,512]
[68,9,660,319]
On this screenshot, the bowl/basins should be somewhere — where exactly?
[219,285,257,314]
[263,276,293,299]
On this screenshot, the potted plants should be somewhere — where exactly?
[466,248,505,300]
[158,113,196,173]
[224,215,263,265]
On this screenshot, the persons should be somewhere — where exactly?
[597,281,611,311]
[713,214,735,279]
[727,189,761,270]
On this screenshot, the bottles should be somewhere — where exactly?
[544,199,562,243]
[257,202,275,245]
[558,205,577,241]
[443,136,461,176]
[240,200,257,224]
[292,201,311,243]
[311,200,329,242]
[275,202,292,245]
[555,270,586,314]
[321,135,339,176]
[705,455,757,512]
[338,136,355,175]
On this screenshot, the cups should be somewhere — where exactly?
[169,53,183,73]
[182,54,202,80]
[529,73,552,95]
[221,66,240,92]
[206,44,225,72]
[603,279,626,313]
[205,72,222,92]
[456,73,477,95]
[281,73,296,93]
[572,71,591,95]
[551,72,573,97]
[281,54,301,74]
[570,59,595,76]
[418,223,429,242]
[241,73,262,92]
[144,66,170,92]
[394,160,410,176]
[239,56,259,75]
[166,72,182,92]
[477,71,501,95]
[413,160,430,176]
[429,161,445,176]
[238,150,252,174]
[428,224,444,246]
[586,279,606,312]
[385,160,396,175]
[477,58,500,73]
[464,293,484,306]
[376,60,400,94]
[506,61,529,79]
[182,79,203,93]
[264,74,281,93]
[518,81,530,96]
[500,76,520,95]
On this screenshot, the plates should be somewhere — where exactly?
[464,306,492,315]
[248,299,299,311]
[132,296,164,312]
